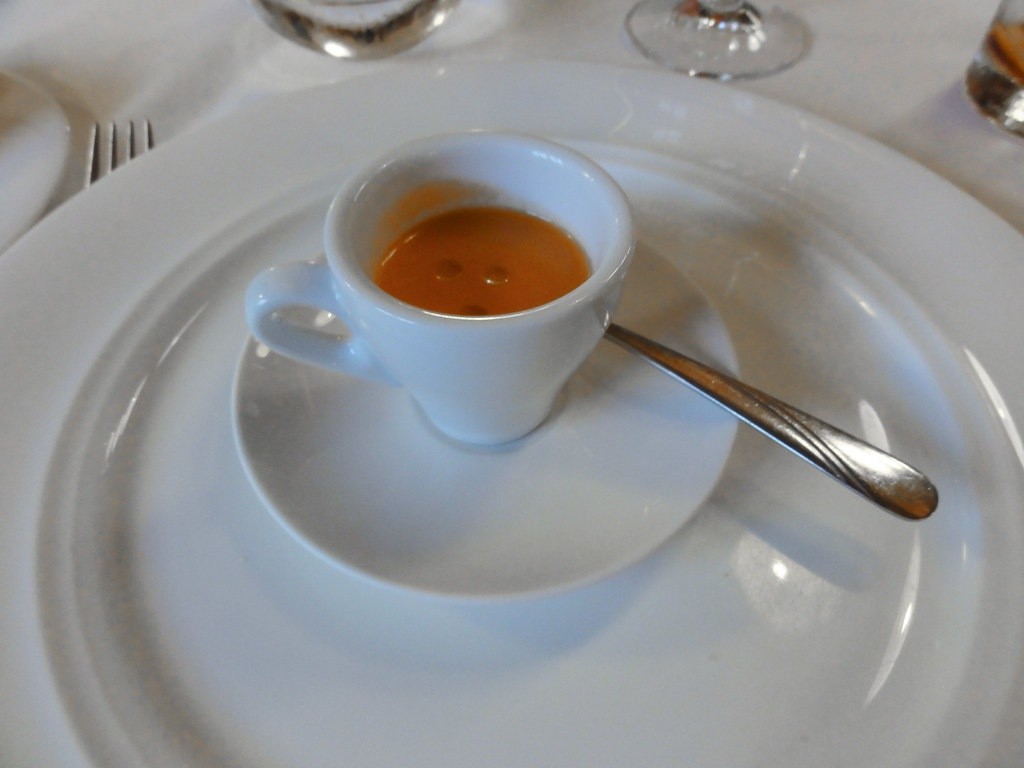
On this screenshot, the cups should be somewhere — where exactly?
[964,0,1024,138]
[254,0,460,62]
[242,132,638,443]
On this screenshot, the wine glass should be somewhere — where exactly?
[625,0,812,81]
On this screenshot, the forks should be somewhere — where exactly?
[86,119,154,187]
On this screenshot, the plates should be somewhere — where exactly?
[1,59,1023,768]
[230,244,741,599]
[1,72,72,255]
